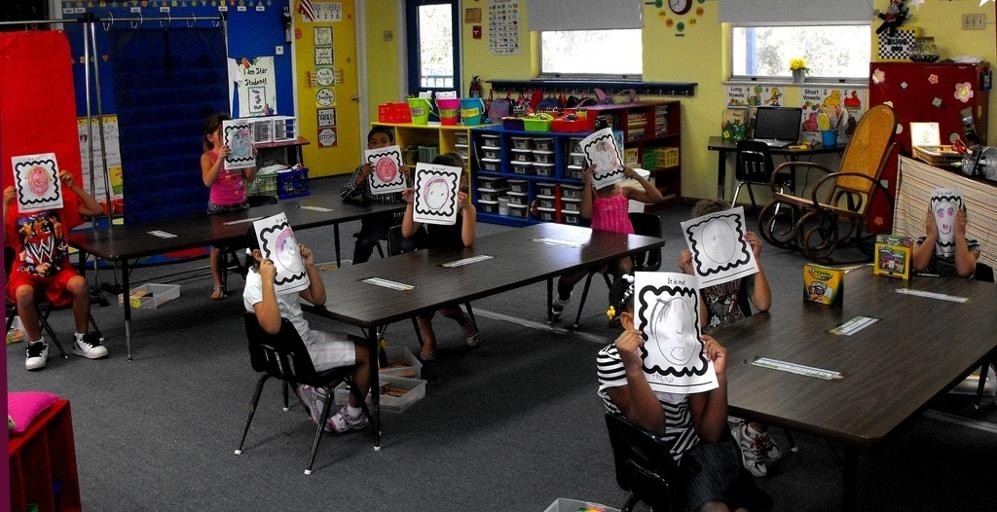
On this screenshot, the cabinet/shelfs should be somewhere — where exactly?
[373,119,471,206]
[865,61,992,235]
[590,100,684,208]
[472,131,621,219]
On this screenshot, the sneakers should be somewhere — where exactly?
[297,384,333,432]
[731,421,782,477]
[466,332,480,347]
[420,352,435,361]
[330,406,368,432]
[72,332,108,359]
[552,291,571,314]
[25,336,49,371]
[211,285,225,299]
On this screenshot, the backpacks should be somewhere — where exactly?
[484,88,614,123]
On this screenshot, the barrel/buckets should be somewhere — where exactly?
[407,97,434,124]
[822,129,836,145]
[435,97,460,126]
[460,97,486,125]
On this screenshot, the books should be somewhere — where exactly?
[621,103,679,174]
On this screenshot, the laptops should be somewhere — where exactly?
[750,107,802,149]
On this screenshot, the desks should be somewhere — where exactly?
[273,218,666,453]
[249,137,316,194]
[699,262,997,512]
[67,192,406,364]
[706,134,848,210]
[5,391,81,511]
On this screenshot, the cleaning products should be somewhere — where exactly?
[817,108,830,132]
[489,98,509,122]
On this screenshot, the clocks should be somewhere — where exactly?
[666,0,691,17]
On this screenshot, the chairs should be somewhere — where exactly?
[1,246,104,358]
[758,105,905,264]
[387,224,478,347]
[601,414,687,511]
[939,258,995,408]
[220,194,279,287]
[570,210,663,331]
[726,141,791,234]
[231,301,377,473]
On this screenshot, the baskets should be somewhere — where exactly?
[654,148,678,169]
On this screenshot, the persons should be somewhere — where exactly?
[678,200,782,476]
[548,124,664,315]
[401,152,480,363]
[0,170,108,371]
[341,127,409,263]
[244,225,372,432]
[199,114,259,300]
[914,197,979,282]
[596,272,736,512]
